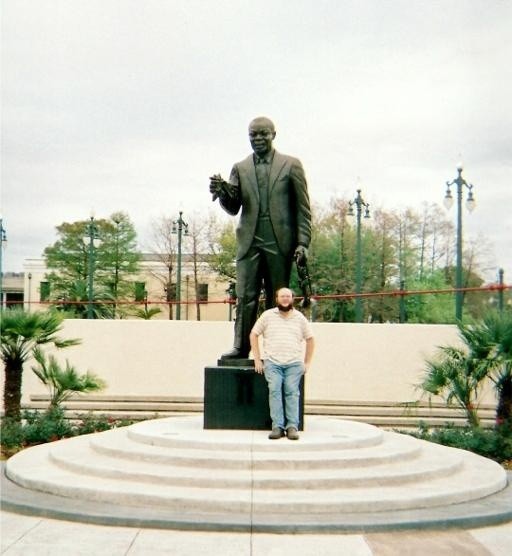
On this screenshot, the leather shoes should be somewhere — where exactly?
[269,427,299,440]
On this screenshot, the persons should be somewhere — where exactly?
[208,117,311,360]
[249,286,314,439]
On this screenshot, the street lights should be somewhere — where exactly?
[0,217,8,313]
[172,210,189,321]
[345,189,371,323]
[27,271,32,315]
[85,216,102,318]
[186,274,189,321]
[443,166,475,323]
